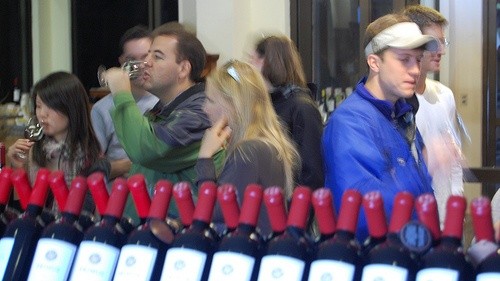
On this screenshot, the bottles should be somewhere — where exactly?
[303,189,362,281]
[218,183,240,240]
[411,195,474,281]
[311,187,339,247]
[203,183,265,281]
[1,144,216,281]
[12,77,20,103]
[267,186,288,243]
[355,192,414,281]
[470,196,500,281]
[415,194,443,249]
[362,193,389,257]
[255,185,312,281]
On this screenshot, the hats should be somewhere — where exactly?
[364,22,439,58]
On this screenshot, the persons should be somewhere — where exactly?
[196,58,302,240]
[253,34,324,192]
[7,71,104,219]
[401,5,463,232]
[321,12,456,244]
[106,22,212,226]
[466,188,500,265]
[90,25,160,195]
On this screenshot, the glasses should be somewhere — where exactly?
[435,36,449,46]
[222,59,242,84]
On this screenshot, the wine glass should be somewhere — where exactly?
[15,117,46,162]
[98,60,144,90]
